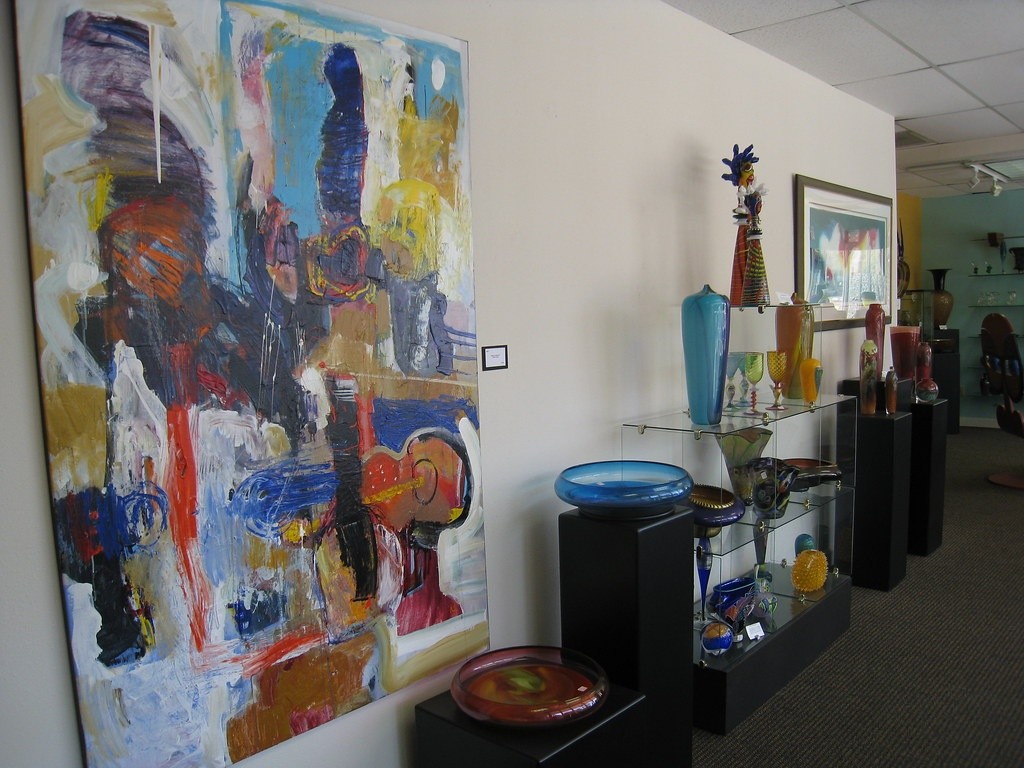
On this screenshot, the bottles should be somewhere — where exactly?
[977,289,1018,306]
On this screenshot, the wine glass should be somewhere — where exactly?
[766,351,787,410]
[722,355,743,411]
[742,351,763,415]
[730,352,758,408]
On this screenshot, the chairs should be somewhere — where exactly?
[979,313,1024,489]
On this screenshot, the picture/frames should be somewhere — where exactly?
[481,343,509,370]
[793,174,893,332]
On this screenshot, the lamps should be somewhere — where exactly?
[968,170,980,188]
[989,179,1003,196]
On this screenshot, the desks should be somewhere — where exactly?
[414,678,646,768]
[836,411,913,593]
[901,399,945,557]
[556,505,695,768]
[933,354,960,434]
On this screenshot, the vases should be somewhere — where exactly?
[743,225,770,305]
[729,225,747,307]
[680,284,731,425]
[926,268,952,330]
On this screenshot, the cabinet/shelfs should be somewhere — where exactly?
[967,236,1024,372]
[619,394,859,736]
